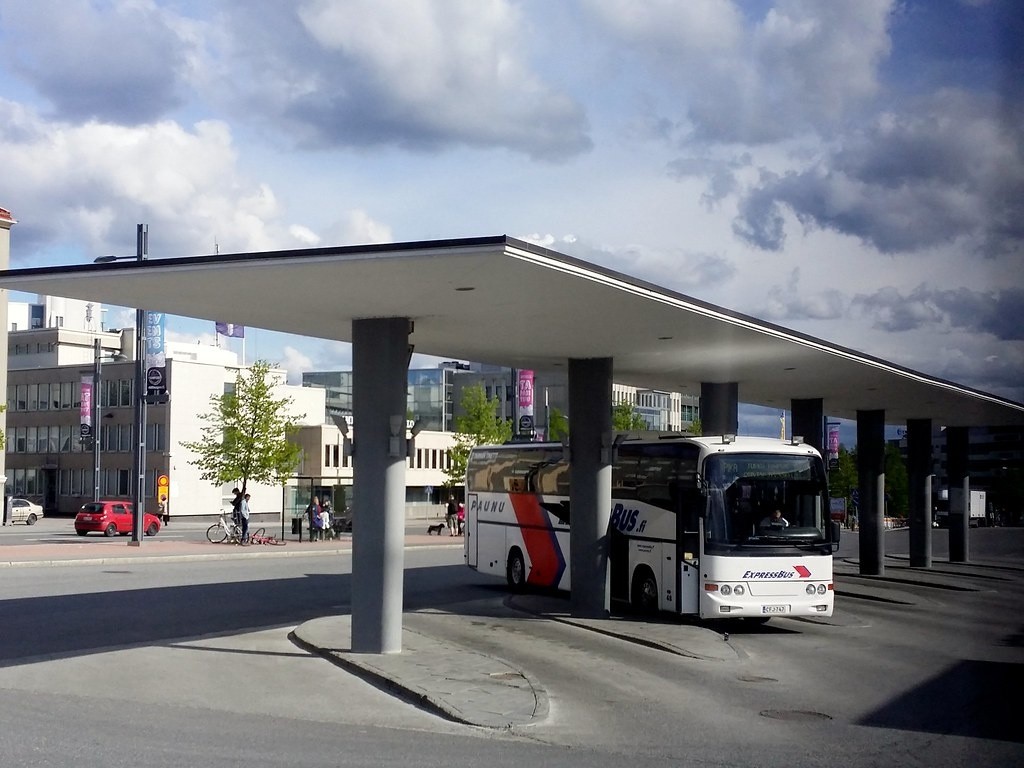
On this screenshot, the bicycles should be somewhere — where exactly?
[249,527,286,546]
[206,508,249,545]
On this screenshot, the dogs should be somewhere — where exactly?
[426,524,445,535]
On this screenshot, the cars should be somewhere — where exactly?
[12,499,44,525]
[75,500,161,537]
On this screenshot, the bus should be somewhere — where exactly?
[463,430,836,624]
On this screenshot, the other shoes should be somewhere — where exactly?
[450,535,453,536]
[455,535,458,536]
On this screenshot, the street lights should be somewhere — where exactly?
[92,338,129,502]
[92,222,150,544]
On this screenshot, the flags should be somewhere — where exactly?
[827,422,842,471]
[216,320,245,340]
[79,370,96,444]
[144,309,169,404]
[516,368,537,438]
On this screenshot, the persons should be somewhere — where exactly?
[302,496,338,544]
[157,496,168,527]
[228,487,242,534]
[240,493,250,543]
[768,507,789,528]
[445,494,466,537]
